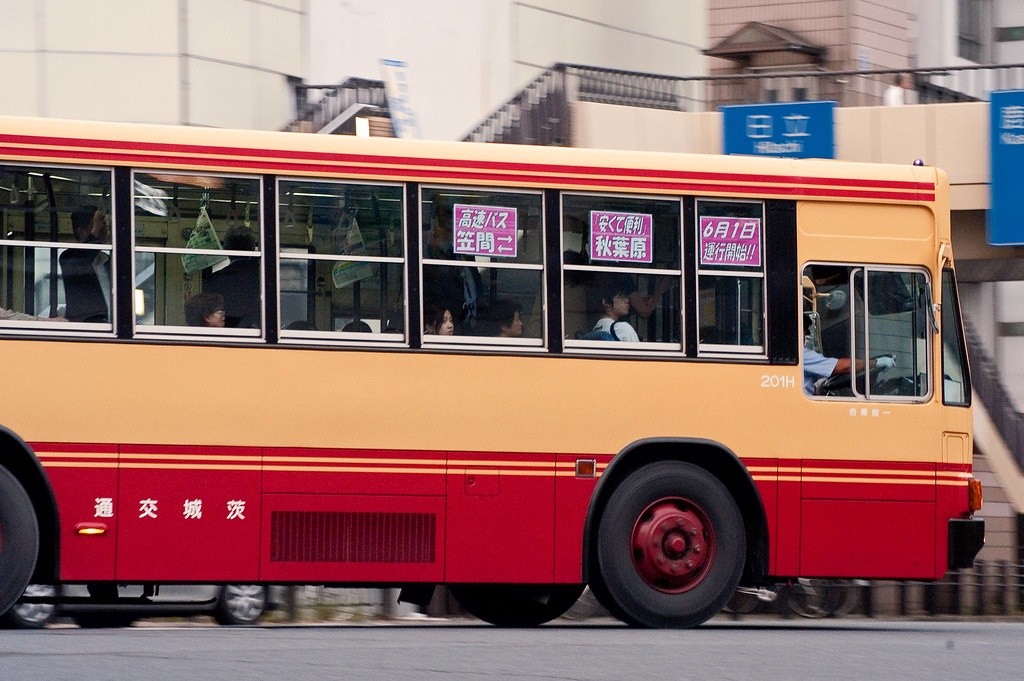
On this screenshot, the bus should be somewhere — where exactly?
[1,115,987,631]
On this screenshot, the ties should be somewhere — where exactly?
[461,267,472,300]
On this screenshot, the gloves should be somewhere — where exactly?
[876,356,896,369]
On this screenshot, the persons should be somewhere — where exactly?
[60,206,113,323]
[803,315,896,395]
[185,207,672,342]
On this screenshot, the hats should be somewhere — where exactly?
[186,293,226,323]
